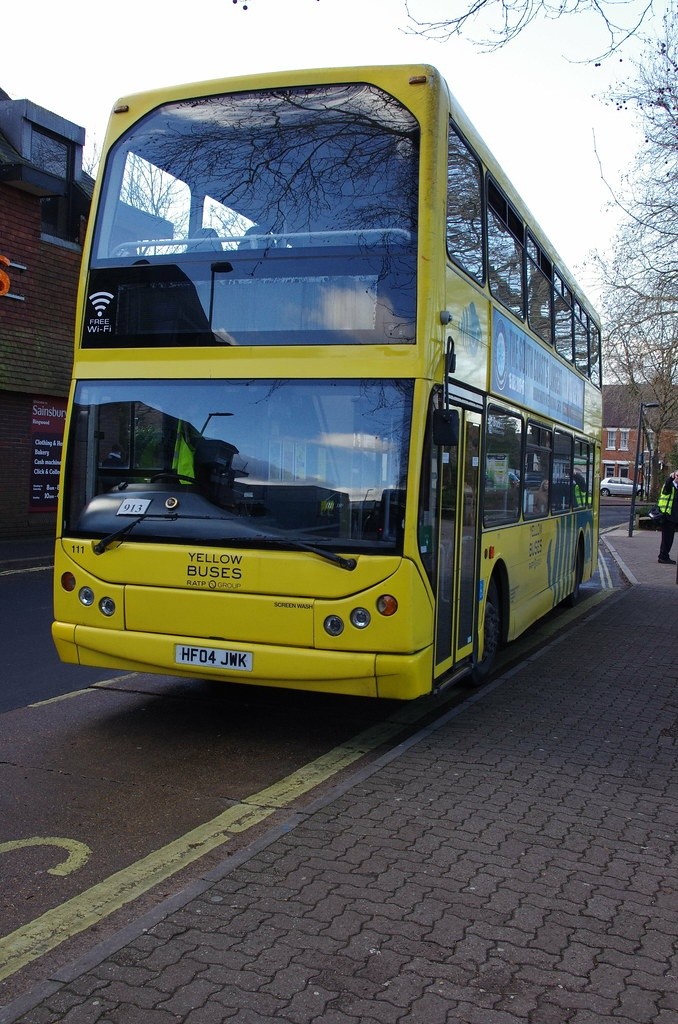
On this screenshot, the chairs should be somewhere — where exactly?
[186,228,222,252]
[238,226,275,250]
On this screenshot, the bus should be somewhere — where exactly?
[51,63,605,700]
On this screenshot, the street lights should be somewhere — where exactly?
[628,403,659,537]
[362,489,374,519]
[193,413,234,445]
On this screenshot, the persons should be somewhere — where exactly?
[573,471,592,507]
[656,470,678,564]
[537,480,548,512]
[101,444,128,493]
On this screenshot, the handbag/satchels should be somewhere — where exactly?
[648,505,664,522]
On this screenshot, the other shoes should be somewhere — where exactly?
[658,557,676,564]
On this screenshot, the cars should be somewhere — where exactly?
[600,477,645,496]
